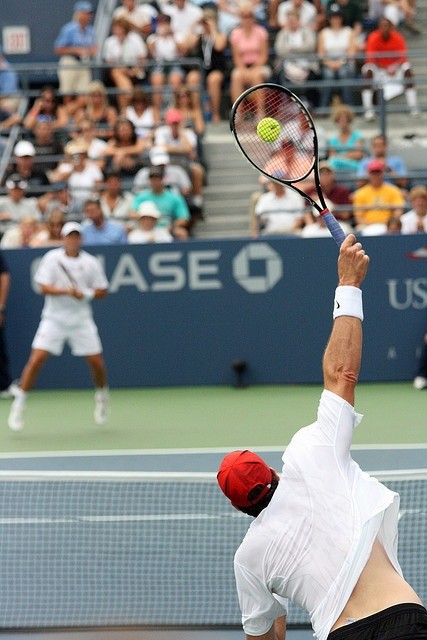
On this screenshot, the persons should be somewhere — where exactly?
[217,234,426,640]
[0,86,206,245]
[251,106,427,238]
[8,221,110,432]
[54,0,419,121]
[0,253,10,400]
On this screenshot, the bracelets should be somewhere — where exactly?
[82,287,94,301]
[332,285,364,321]
[0,305,7,314]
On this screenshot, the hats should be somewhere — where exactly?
[13,141,35,157]
[49,181,68,192]
[165,108,181,126]
[409,186,426,202]
[6,174,28,190]
[216,450,272,506]
[316,161,333,173]
[327,3,342,15]
[282,138,295,145]
[60,222,85,237]
[148,146,169,166]
[137,201,160,221]
[368,161,385,173]
[73,0,94,13]
[258,160,288,183]
[149,166,165,178]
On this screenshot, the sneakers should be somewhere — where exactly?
[411,108,420,116]
[414,376,427,390]
[7,390,25,430]
[365,109,376,121]
[403,22,422,35]
[93,385,110,426]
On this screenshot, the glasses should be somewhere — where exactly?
[177,95,190,101]
[6,182,29,191]
[92,93,103,98]
[37,115,56,124]
[41,96,53,105]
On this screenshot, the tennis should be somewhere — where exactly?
[257,118,280,142]
